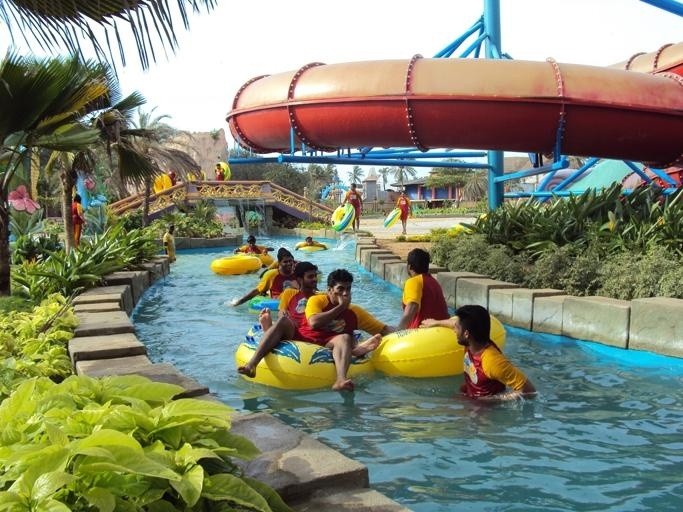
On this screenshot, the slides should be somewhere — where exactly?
[227,40,683,189]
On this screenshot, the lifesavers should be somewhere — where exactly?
[383,206,402,229]
[248,294,280,315]
[210,254,262,275]
[153,174,172,193]
[236,251,273,266]
[330,202,355,233]
[215,161,231,181]
[371,311,506,378]
[234,325,372,390]
[296,241,327,253]
[187,169,206,182]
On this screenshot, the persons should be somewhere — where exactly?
[416,303,538,402]
[341,183,364,235]
[258,261,382,357]
[71,194,86,249]
[162,223,176,264]
[258,246,289,278]
[191,166,201,185]
[394,191,413,235]
[214,163,225,182]
[233,235,275,255]
[236,268,402,392]
[167,168,177,186]
[137,186,156,209]
[395,245,451,331]
[227,251,300,308]
[294,236,329,252]
